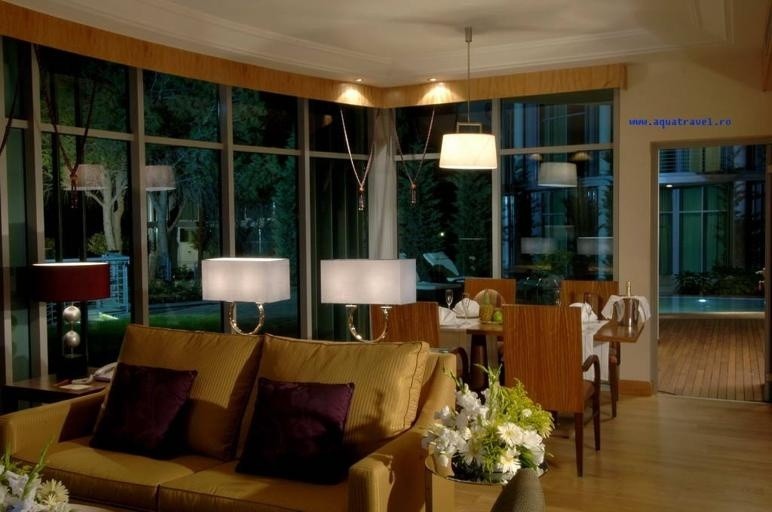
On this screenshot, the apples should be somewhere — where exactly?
[493,311,503,322]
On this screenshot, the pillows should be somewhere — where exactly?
[91,355,195,460]
[233,378,354,488]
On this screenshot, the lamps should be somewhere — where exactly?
[199,257,419,345]
[437,24,499,170]
[19,260,109,381]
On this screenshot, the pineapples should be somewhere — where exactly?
[479,291,493,322]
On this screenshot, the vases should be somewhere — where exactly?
[422,444,504,512]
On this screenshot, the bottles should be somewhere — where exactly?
[625,279,633,296]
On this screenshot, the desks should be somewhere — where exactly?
[0,367,112,411]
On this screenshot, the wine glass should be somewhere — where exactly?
[551,286,599,334]
[443,290,472,327]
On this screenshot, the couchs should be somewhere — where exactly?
[0,323,457,510]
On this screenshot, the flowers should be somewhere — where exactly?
[1,432,75,511]
[422,359,556,485]
